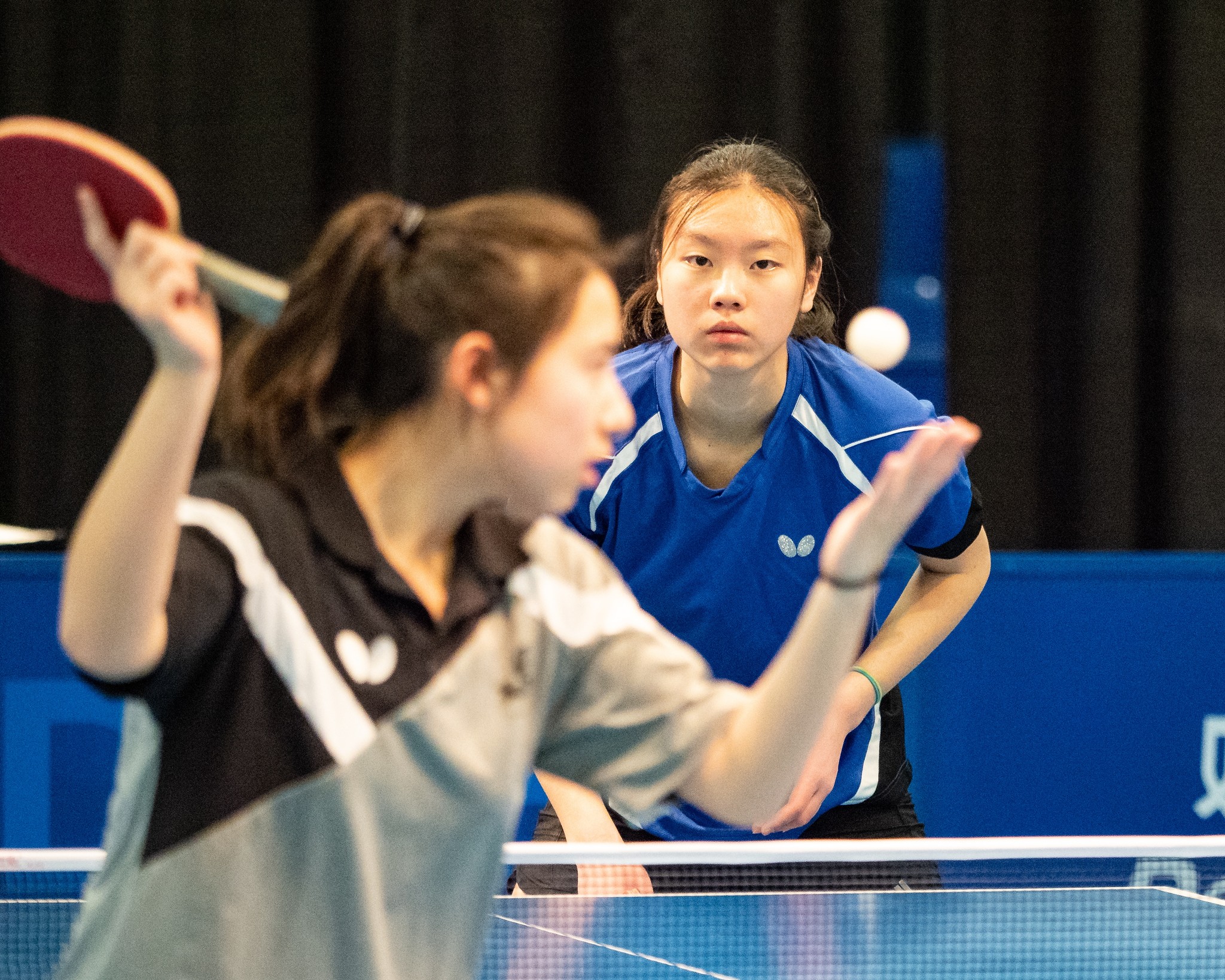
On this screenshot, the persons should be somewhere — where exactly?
[57,192,983,980]
[509,131,991,898]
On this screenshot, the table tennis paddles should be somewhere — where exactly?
[2,113,293,331]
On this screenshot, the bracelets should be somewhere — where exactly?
[849,666,882,705]
[819,568,883,591]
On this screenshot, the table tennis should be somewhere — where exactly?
[844,306,910,373]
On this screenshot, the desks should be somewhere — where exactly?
[0,886,1225,980]
